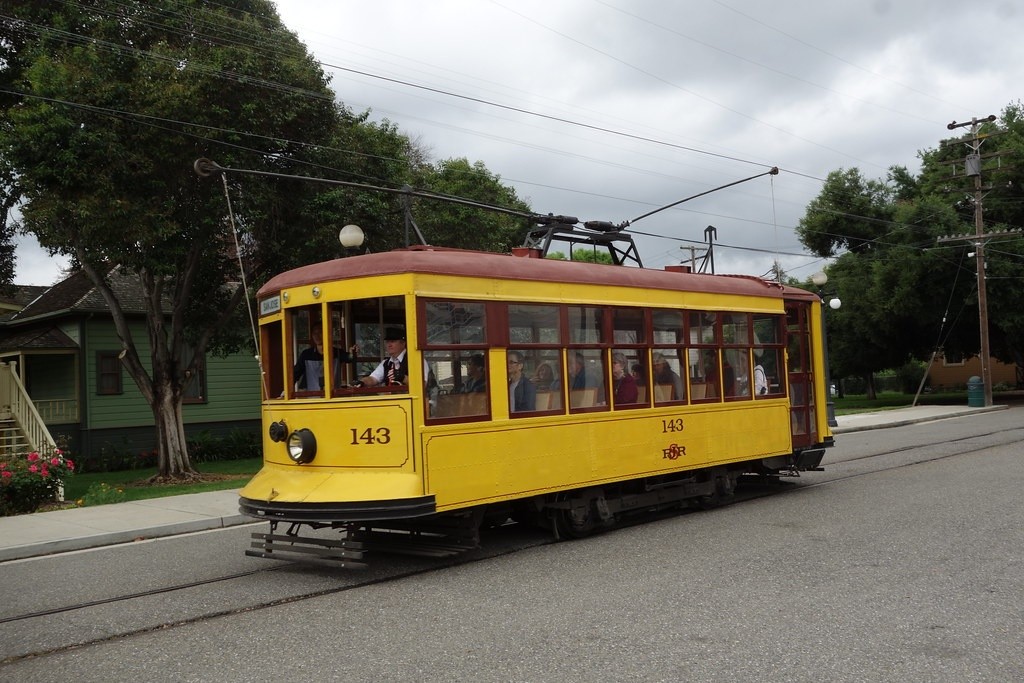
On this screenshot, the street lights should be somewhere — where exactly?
[339,224,364,257]
[806,270,841,427]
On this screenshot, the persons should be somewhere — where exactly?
[650,352,683,400]
[705,349,734,398]
[277,319,360,401]
[735,349,768,397]
[597,353,638,407]
[508,350,536,413]
[350,326,429,394]
[528,362,555,390]
[454,353,487,394]
[549,351,601,390]
[629,364,646,385]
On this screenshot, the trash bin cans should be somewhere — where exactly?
[966,375,985,407]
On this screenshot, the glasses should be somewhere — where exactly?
[507,360,520,364]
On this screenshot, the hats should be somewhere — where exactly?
[652,352,667,364]
[382,327,406,340]
[705,349,715,355]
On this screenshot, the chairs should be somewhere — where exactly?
[433,382,719,417]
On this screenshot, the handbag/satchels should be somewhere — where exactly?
[760,387,766,394]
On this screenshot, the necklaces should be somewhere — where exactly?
[615,374,625,395]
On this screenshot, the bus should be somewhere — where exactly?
[193,134,835,569]
